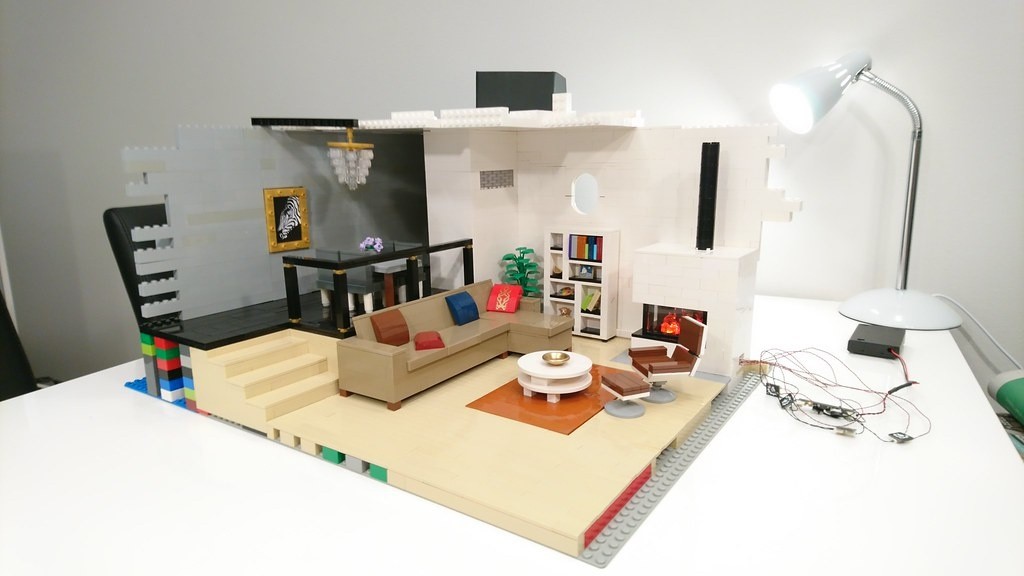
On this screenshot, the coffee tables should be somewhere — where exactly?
[518,350,593,402]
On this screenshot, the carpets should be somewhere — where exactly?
[465,363,631,436]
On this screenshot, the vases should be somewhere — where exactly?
[366,246,374,250]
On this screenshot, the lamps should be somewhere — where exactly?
[327,128,374,191]
[767,54,965,331]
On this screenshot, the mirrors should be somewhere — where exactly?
[571,173,597,213]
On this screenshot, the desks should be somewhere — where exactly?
[0,271,1024,576]
[282,238,474,339]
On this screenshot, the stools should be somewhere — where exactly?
[600,373,651,418]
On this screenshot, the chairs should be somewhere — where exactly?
[627,316,707,404]
[314,242,424,314]
[102,204,181,328]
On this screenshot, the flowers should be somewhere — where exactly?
[359,237,383,253]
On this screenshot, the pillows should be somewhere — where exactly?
[415,331,445,351]
[445,291,479,326]
[487,284,522,313]
[370,309,409,347]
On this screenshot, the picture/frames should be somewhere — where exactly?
[264,188,311,253]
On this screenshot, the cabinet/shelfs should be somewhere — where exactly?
[543,229,621,342]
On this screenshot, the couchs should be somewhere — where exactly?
[337,279,574,411]
